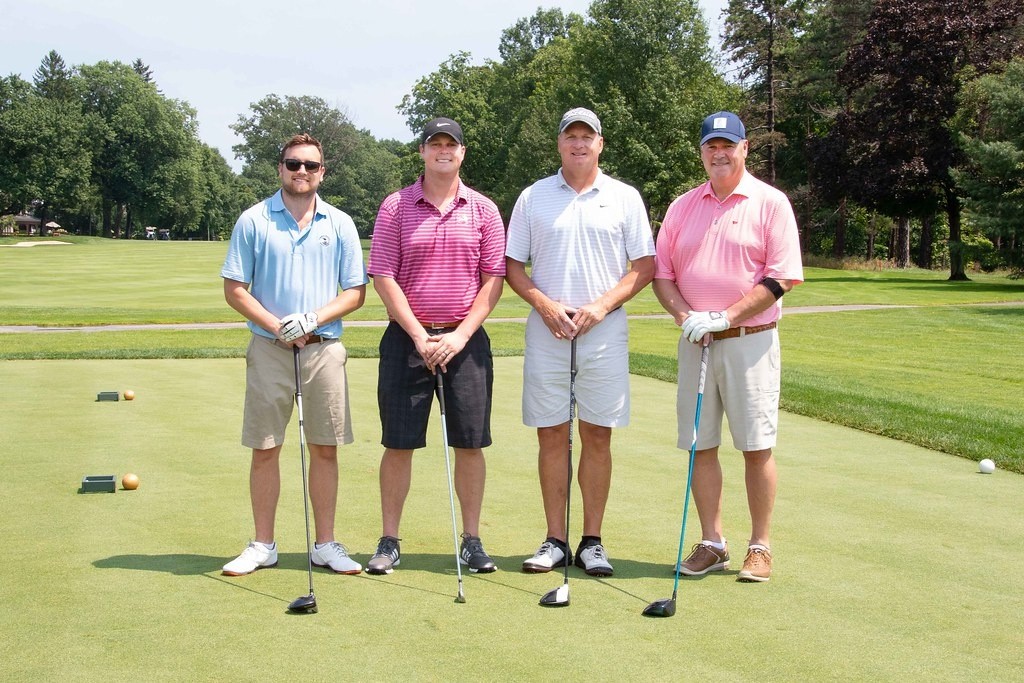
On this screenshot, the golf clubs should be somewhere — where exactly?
[286,343,316,610]
[436,364,467,603]
[641,343,709,618]
[539,314,571,608]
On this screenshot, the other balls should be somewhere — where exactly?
[124,391,134,400]
[122,473,139,490]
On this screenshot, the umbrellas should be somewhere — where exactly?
[46,222,61,228]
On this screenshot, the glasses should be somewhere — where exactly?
[281,158,322,174]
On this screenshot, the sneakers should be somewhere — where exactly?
[573,544,613,575]
[311,542,362,574]
[522,540,573,571]
[223,540,278,576]
[737,544,772,581]
[366,536,401,573]
[457,532,497,573]
[674,540,730,575]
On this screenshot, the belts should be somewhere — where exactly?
[303,335,329,345]
[567,303,623,320]
[389,317,465,330]
[707,323,775,340]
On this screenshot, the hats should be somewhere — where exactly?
[557,107,602,135]
[422,118,464,146]
[699,112,745,143]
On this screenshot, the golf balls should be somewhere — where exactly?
[979,458,995,473]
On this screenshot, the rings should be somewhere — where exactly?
[443,352,448,356]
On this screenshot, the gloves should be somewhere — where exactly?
[681,311,729,342]
[279,312,320,343]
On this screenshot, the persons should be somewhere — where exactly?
[652,112,803,582]
[505,107,657,575]
[367,118,505,573]
[220,135,371,575]
